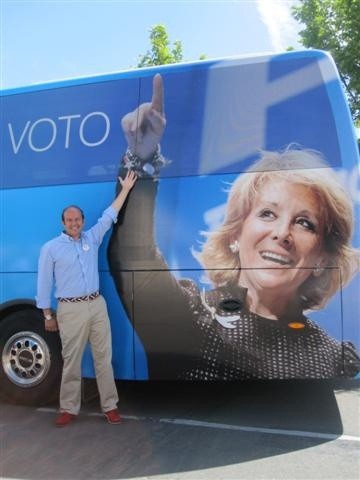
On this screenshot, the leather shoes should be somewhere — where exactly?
[103,409,121,424]
[55,413,80,425]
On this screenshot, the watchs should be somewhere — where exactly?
[43,314,55,320]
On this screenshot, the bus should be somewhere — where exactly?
[0,49,360,407]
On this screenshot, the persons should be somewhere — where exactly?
[34,171,138,427]
[106,72,360,378]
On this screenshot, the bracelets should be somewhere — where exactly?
[122,144,165,177]
